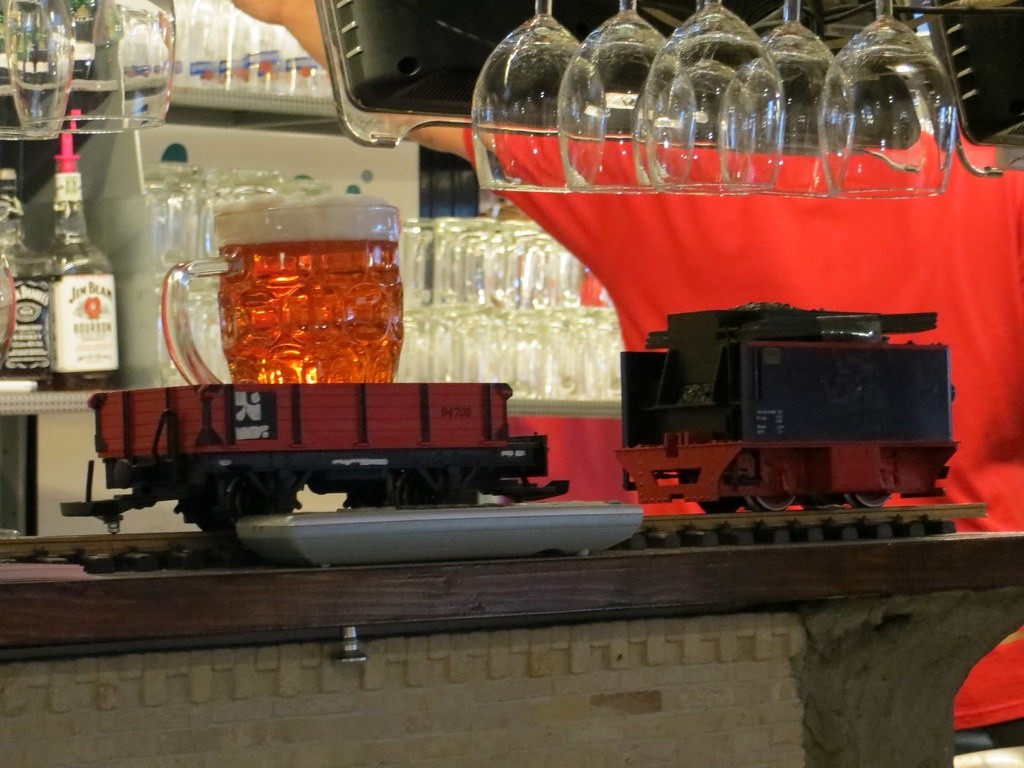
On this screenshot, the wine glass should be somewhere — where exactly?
[471,0,958,200]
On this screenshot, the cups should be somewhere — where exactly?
[0,1,175,141]
[145,163,626,402]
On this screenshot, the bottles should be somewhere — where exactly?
[52,132,120,391]
[0,168,53,390]
